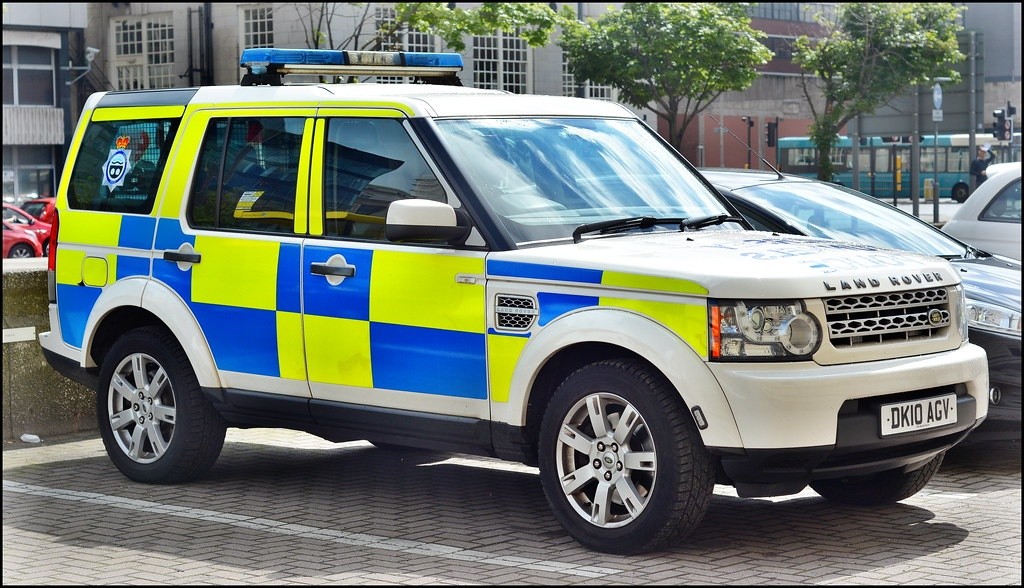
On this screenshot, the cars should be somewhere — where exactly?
[1,197,57,258]
[502,165,1023,474]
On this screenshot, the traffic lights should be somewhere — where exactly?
[993,110,1002,140]
[1005,118,1012,141]
[764,124,768,143]
[1010,105,1017,116]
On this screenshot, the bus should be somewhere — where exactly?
[775,135,994,205]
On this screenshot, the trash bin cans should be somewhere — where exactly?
[923,178,935,201]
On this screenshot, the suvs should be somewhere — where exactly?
[31,45,988,556]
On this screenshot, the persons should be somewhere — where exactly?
[969,144,996,189]
